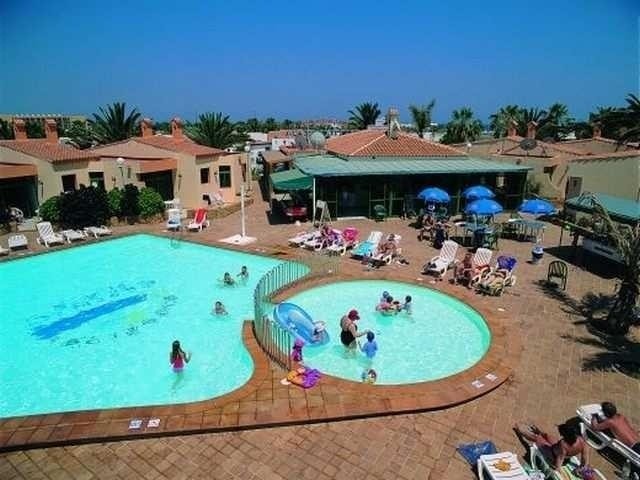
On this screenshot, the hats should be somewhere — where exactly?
[350,310,360,320]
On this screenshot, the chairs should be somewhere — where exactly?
[166,208,210,232]
[475,403,640,480]
[289,204,568,296]
[0,207,112,254]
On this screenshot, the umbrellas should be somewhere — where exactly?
[416,187,450,214]
[461,186,498,199]
[461,199,504,226]
[517,198,561,221]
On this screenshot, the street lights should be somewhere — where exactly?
[244,141,251,191]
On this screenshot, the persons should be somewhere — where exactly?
[374,290,413,318]
[169,339,193,372]
[357,330,379,359]
[519,418,590,476]
[311,320,327,343]
[288,338,304,365]
[307,212,510,290]
[590,399,639,456]
[363,370,376,384]
[339,309,370,353]
[217,272,236,286]
[236,264,249,281]
[211,302,229,315]
